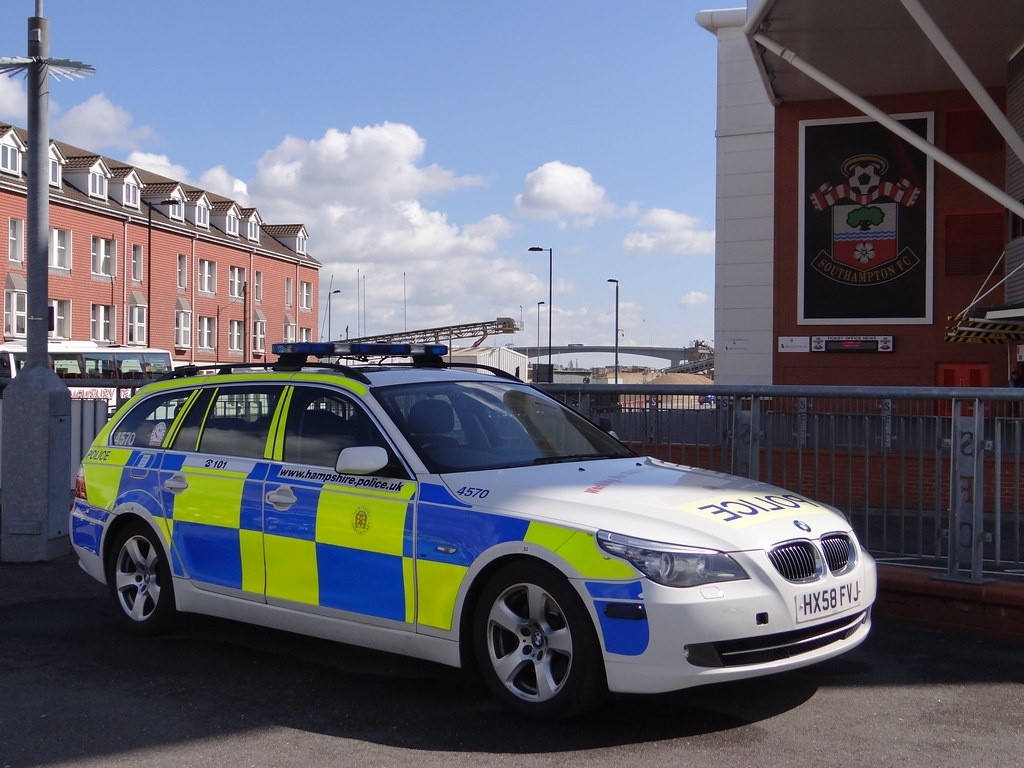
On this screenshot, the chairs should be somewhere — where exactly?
[162,397,478,477]
[55,367,169,379]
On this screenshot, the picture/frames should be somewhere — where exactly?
[797,110,934,326]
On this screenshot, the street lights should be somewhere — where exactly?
[536,301,545,382]
[529,244,556,383]
[606,277,621,384]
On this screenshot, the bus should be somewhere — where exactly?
[0,331,174,424]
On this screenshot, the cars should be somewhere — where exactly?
[64,333,884,727]
